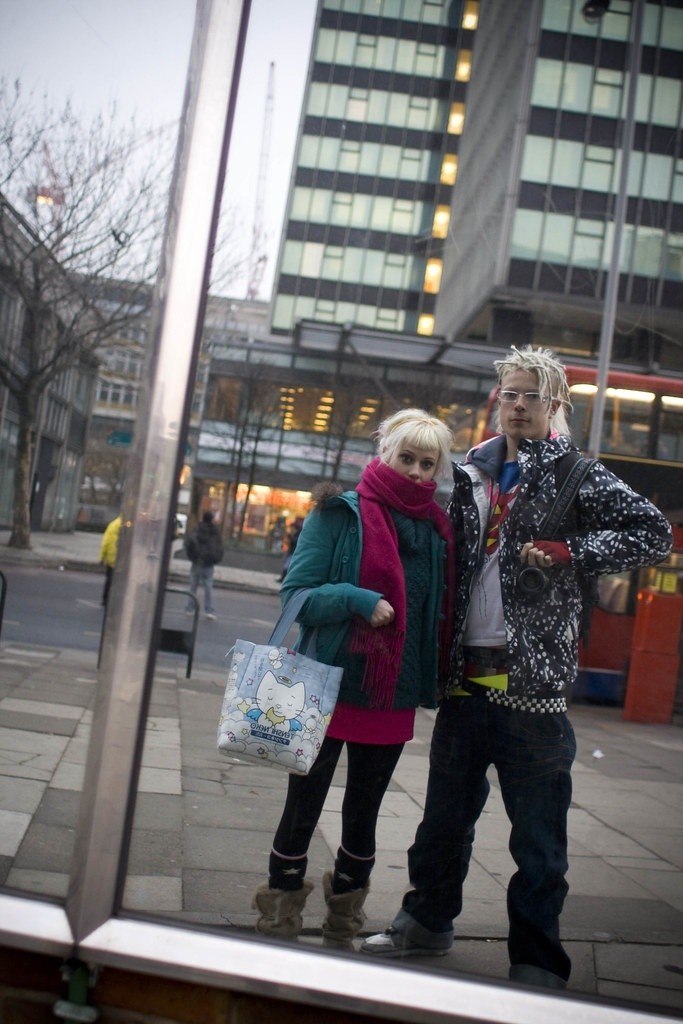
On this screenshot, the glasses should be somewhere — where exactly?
[497,389,548,406]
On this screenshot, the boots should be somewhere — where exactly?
[321,872,368,951]
[251,882,313,942]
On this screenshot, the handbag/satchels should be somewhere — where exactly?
[217,588,353,776]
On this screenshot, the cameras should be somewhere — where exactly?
[514,555,553,597]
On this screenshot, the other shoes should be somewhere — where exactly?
[206,614,216,619]
[187,610,195,614]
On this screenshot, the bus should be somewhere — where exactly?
[469,367,682,709]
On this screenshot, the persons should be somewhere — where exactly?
[248,409,458,949]
[183,511,223,620]
[360,342,674,992]
[98,511,121,605]
[269,512,306,583]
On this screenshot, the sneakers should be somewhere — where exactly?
[360,926,452,957]
[508,961,567,989]
